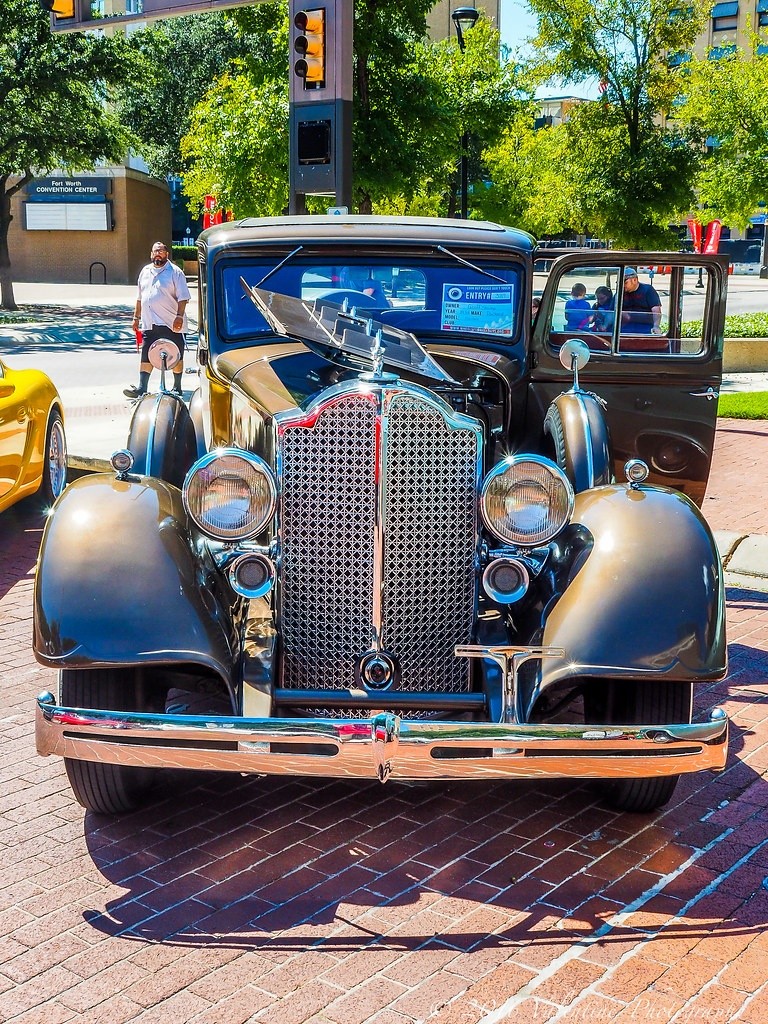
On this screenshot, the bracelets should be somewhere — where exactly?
[133,316,139,320]
[177,314,183,319]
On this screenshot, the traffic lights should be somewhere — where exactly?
[39,0,76,19]
[292,7,325,83]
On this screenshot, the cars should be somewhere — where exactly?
[30,214,730,817]
[1,361,67,513]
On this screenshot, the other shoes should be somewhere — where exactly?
[123,384,142,398]
[171,388,182,395]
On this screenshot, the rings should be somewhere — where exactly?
[178,327,180,328]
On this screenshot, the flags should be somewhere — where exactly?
[598,75,608,93]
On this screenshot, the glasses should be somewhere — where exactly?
[153,249,167,255]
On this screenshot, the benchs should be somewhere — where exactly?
[379,309,439,331]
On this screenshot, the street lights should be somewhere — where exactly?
[450,6,480,221]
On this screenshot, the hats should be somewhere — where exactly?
[624,268,638,275]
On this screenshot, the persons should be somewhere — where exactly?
[123,241,188,398]
[564,268,662,334]
[339,266,390,309]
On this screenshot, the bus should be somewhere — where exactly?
[677,238,765,276]
